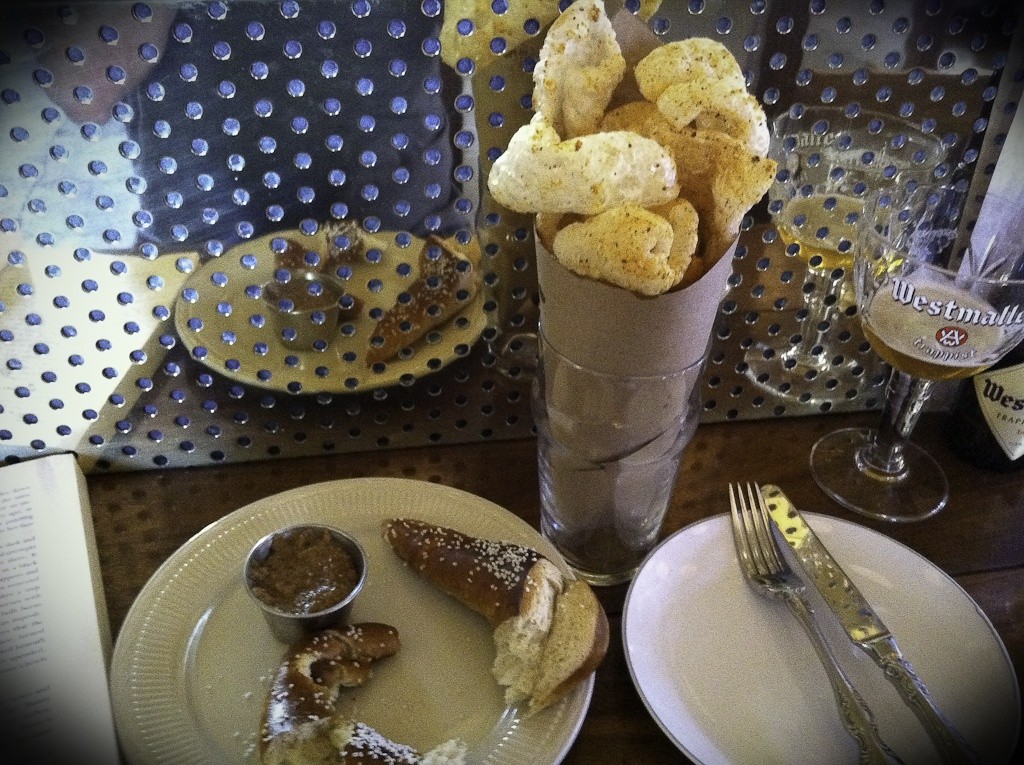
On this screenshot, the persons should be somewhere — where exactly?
[0,0,481,256]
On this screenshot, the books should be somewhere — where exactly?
[0,452,121,765]
[0,234,202,471]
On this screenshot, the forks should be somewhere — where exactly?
[729,480,904,765]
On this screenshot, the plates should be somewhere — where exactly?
[108,476,597,765]
[620,511,1022,765]
[172,220,488,394]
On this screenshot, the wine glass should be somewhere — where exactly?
[740,104,952,404]
[806,183,1023,525]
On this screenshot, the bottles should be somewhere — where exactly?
[943,254,1024,474]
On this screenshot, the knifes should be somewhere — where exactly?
[758,482,981,765]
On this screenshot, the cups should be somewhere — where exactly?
[242,523,369,645]
[533,319,714,588]
[261,270,345,351]
[473,158,538,383]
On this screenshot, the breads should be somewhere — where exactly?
[256,619,467,765]
[272,218,387,274]
[383,518,610,713]
[366,236,477,367]
